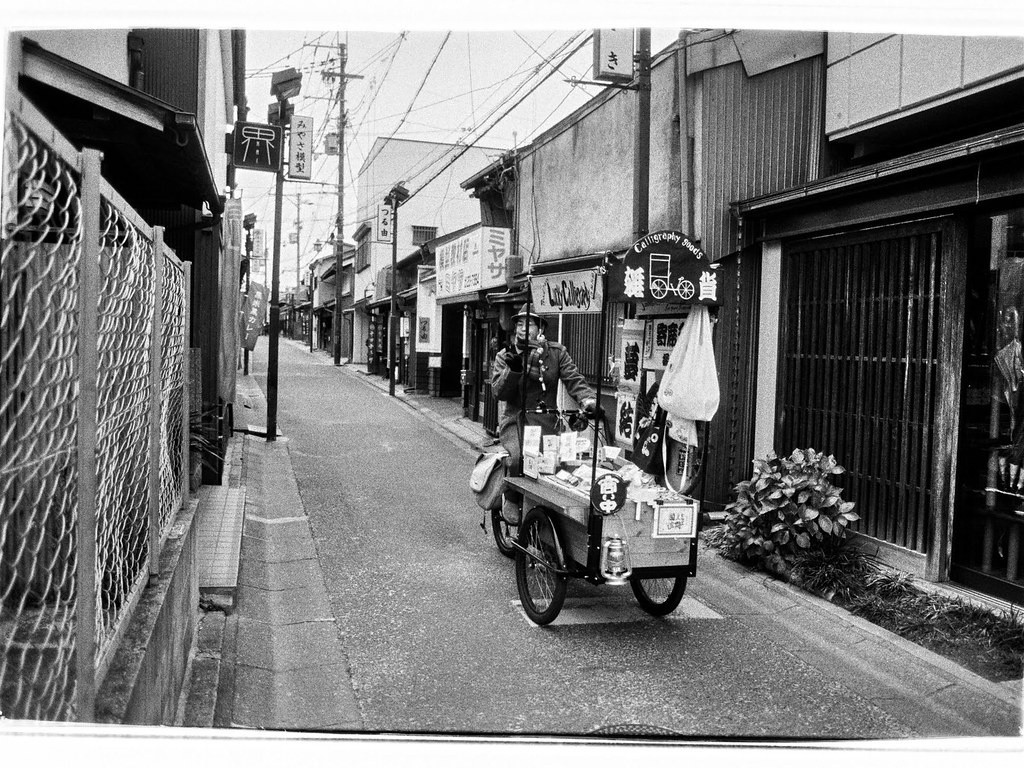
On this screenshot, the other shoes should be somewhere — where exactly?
[502,499,519,523]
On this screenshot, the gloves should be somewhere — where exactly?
[583,403,606,420]
[502,341,524,372]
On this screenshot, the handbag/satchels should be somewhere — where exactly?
[665,411,698,446]
[470,451,508,511]
[657,304,720,421]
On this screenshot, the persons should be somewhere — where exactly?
[491,302,605,526]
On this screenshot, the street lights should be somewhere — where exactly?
[242,212,257,374]
[387,182,409,396]
[266,66,303,441]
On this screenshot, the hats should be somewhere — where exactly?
[510,303,549,332]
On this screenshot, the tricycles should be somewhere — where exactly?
[477,231,725,625]
